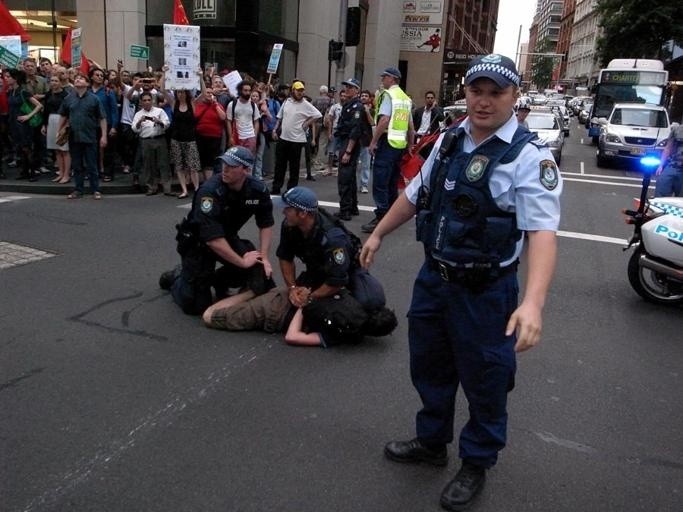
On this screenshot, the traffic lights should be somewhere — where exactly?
[332,42,343,60]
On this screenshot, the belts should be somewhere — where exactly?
[426,254,511,282]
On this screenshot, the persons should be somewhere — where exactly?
[159,145,274,315]
[360,53,563,512]
[272,186,386,308]
[654,121,683,197]
[516,103,531,130]
[203,237,398,350]
[417,29,441,52]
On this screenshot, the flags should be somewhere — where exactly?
[173,0,190,24]
[0,0,32,42]
[62,31,89,73]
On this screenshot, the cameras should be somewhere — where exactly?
[142,79,153,84]
[145,116,154,121]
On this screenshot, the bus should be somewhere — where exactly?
[588,57,672,144]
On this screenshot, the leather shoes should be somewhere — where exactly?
[0,157,189,199]
[439,467,486,512]
[264,167,381,233]
[159,263,182,290]
[384,437,449,466]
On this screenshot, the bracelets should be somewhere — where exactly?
[307,292,317,305]
[287,281,297,288]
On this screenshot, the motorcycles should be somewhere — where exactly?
[621,153,683,306]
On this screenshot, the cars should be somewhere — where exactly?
[440,87,672,174]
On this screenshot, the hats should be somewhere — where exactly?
[215,146,255,168]
[464,53,520,89]
[518,102,531,111]
[292,81,305,90]
[380,66,401,79]
[341,78,361,89]
